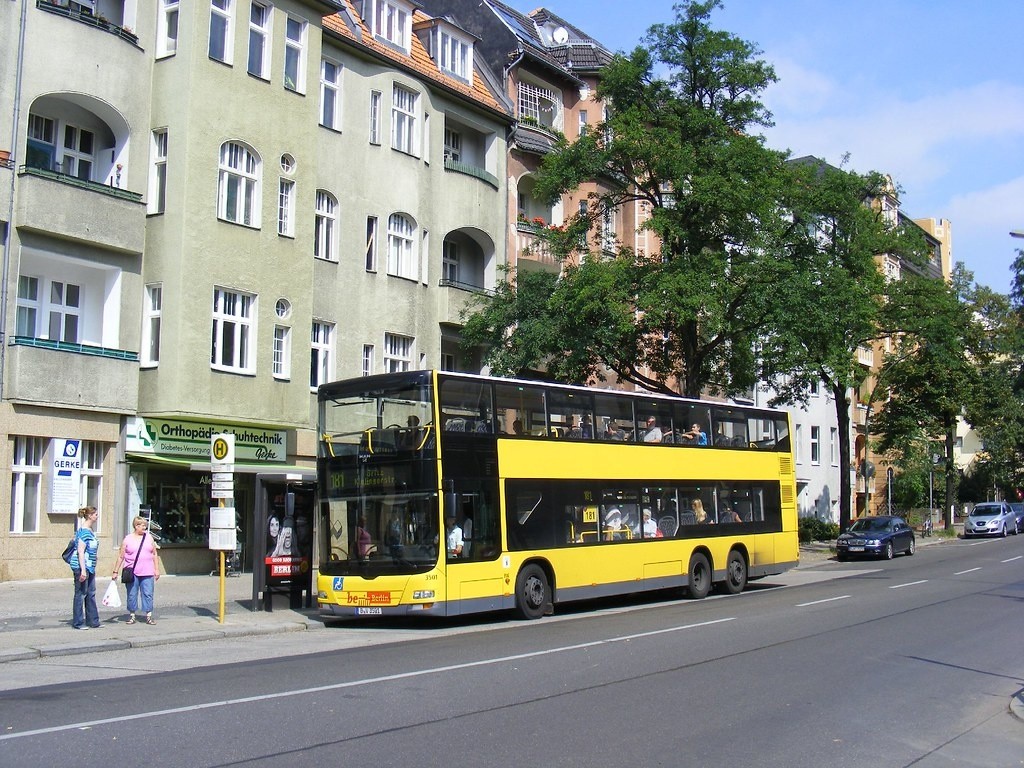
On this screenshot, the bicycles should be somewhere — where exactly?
[921,523,932,539]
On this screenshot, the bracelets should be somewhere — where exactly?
[113,571,118,573]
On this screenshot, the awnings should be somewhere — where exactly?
[125,451,317,473]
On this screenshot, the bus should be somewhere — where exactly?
[315,368,802,621]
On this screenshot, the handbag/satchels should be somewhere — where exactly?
[61,539,77,564]
[121,568,134,583]
[101,580,121,608]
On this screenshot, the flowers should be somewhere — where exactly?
[517,210,562,233]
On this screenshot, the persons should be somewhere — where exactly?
[691,499,713,524]
[640,416,672,443]
[433,518,465,559]
[581,415,592,439]
[356,518,371,558]
[466,419,502,434]
[268,516,309,556]
[712,422,722,444]
[719,501,741,522]
[71,507,105,629]
[601,495,656,540]
[401,416,421,450]
[112,517,159,625]
[662,501,676,518]
[682,423,708,445]
[513,420,524,436]
[387,511,403,550]
[604,422,622,440]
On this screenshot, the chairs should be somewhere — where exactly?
[461,516,473,558]
[365,418,760,452]
[621,497,761,537]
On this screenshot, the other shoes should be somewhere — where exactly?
[80,625,90,630]
[98,624,105,628]
[126,614,136,624]
[146,617,156,625]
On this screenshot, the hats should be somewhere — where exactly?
[643,508,652,516]
[283,517,295,527]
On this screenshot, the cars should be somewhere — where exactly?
[836,514,916,560]
[999,502,1024,533]
[963,500,1019,539]
